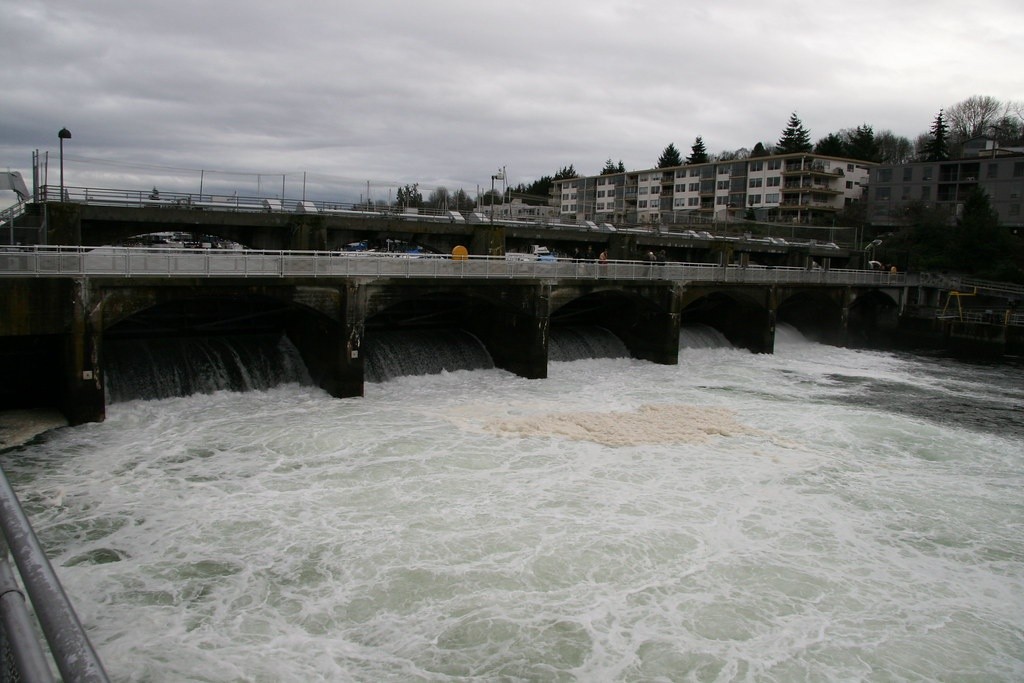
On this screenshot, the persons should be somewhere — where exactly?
[572,248,582,263]
[211,237,218,249]
[879,261,897,280]
[642,248,658,265]
[599,248,608,264]
[585,245,596,263]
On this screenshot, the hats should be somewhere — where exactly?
[648,252,653,255]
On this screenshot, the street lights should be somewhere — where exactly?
[490,173,506,224]
[57,127,74,200]
